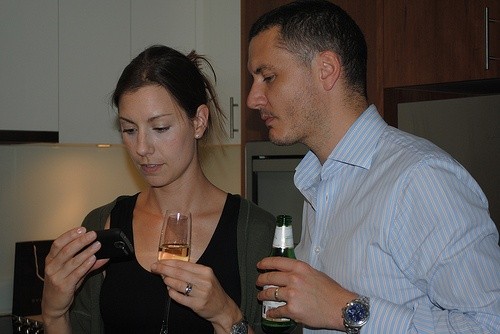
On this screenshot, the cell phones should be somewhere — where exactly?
[73,229,133,261]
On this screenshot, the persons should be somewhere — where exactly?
[246,0,500,334]
[40,44,277,334]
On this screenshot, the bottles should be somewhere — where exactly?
[261,214,298,334]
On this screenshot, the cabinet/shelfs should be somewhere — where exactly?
[0,0,500,149]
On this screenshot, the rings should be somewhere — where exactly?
[275,288,280,301]
[184,284,192,296]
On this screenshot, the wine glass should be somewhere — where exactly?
[156,208,192,334]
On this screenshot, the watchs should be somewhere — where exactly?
[214,312,248,334]
[341,295,370,334]
